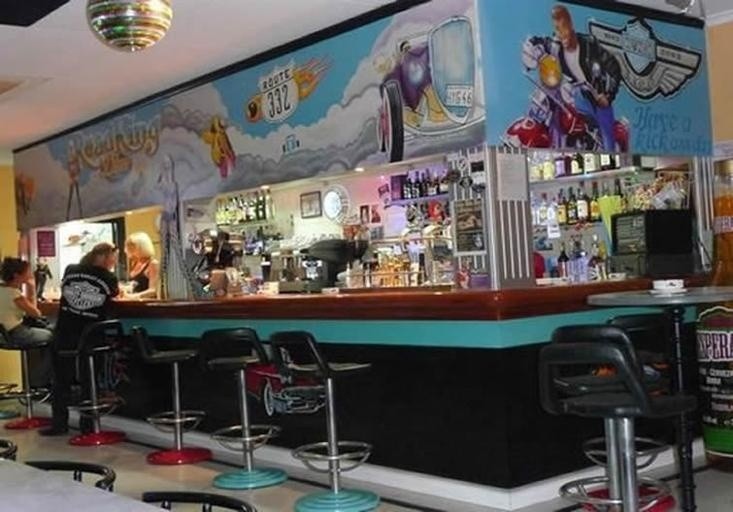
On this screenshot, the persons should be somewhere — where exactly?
[65,137,84,220]
[37,243,124,435]
[124,232,159,299]
[522,6,622,152]
[0,256,56,379]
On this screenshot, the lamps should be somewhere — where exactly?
[86,0,175,53]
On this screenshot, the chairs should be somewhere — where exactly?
[537,311,699,512]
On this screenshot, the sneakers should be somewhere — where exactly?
[36,426,67,437]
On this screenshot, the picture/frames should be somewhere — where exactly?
[299,190,322,219]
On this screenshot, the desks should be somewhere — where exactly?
[587,287,733,512]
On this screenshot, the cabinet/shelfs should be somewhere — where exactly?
[341,164,452,291]
[530,166,637,230]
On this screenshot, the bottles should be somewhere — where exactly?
[346,241,442,288]
[694,158,732,474]
[527,153,639,281]
[401,165,451,199]
[214,190,277,224]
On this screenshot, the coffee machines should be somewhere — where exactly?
[277,238,351,294]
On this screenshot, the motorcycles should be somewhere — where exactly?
[495,43,631,153]
